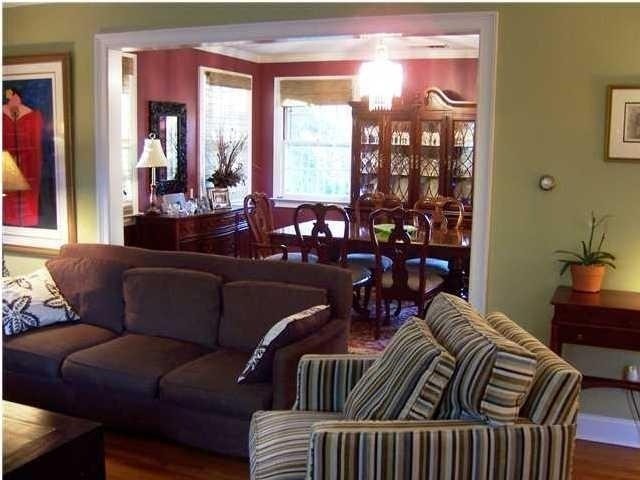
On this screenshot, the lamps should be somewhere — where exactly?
[137,132,168,216]
[354,40,404,111]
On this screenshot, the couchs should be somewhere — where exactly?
[3,244,352,460]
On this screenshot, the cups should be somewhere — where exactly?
[185,201,196,215]
[169,203,181,215]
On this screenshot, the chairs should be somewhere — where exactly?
[250,293,581,478]
[244,191,471,340]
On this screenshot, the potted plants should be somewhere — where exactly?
[551,211,617,294]
[205,128,249,210]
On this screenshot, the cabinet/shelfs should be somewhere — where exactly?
[134,204,250,260]
[347,87,477,230]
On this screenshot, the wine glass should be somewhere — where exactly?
[364,127,378,143]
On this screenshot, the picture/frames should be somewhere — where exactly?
[205,184,231,209]
[2,54,77,257]
[604,84,640,160]
[148,101,187,195]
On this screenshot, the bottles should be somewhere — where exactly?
[392,130,474,147]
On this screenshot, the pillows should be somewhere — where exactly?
[239,304,333,383]
[339,318,454,423]
[3,266,79,337]
[425,292,537,421]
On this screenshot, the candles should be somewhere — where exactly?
[189,187,194,199]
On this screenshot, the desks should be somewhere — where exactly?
[549,287,640,396]
